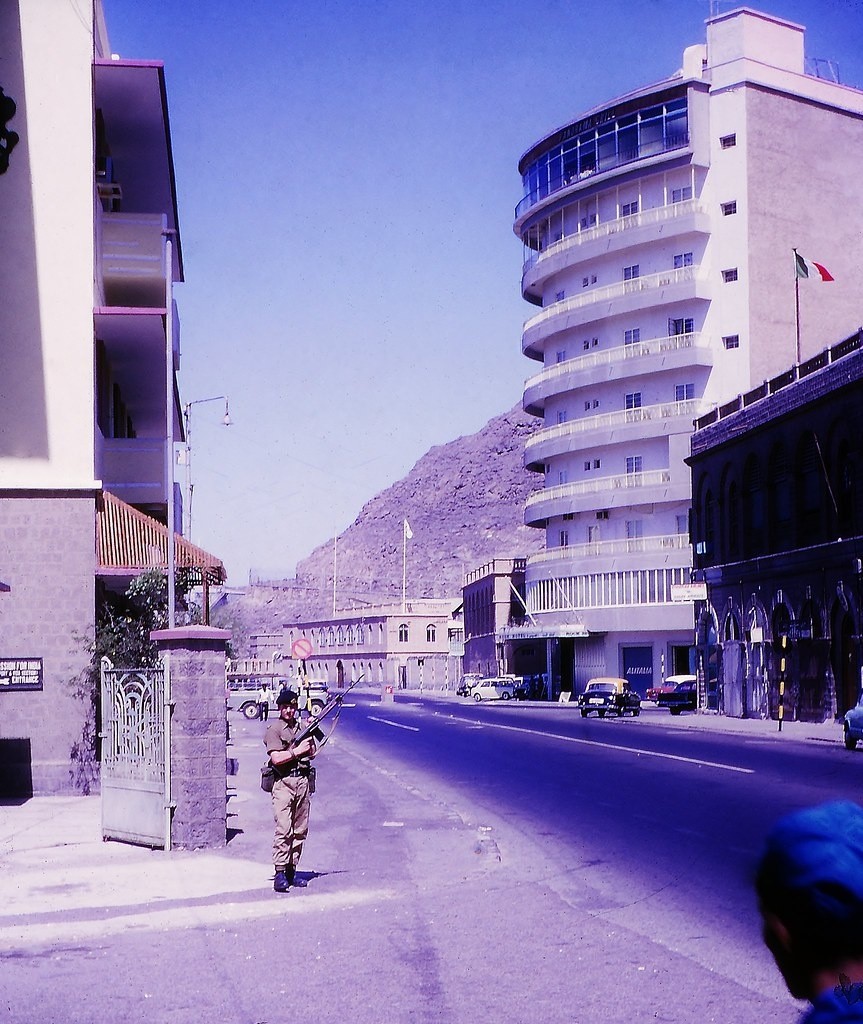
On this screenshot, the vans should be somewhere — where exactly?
[456,672,561,702]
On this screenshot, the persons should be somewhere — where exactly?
[514,672,543,700]
[263,691,316,890]
[256,681,290,722]
[756,800,863,1024]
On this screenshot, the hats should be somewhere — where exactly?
[261,681,268,687]
[276,690,298,705]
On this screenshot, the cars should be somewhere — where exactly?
[576,677,641,717]
[646,674,719,715]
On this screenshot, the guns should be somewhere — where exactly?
[268,673,367,777]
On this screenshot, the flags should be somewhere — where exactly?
[796,253,835,282]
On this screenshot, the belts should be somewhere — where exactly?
[281,769,310,777]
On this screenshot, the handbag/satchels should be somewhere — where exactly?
[260,767,275,792]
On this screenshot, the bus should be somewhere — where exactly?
[227,672,329,720]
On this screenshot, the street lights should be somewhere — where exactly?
[185,395,233,542]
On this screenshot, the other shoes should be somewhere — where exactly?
[307,696,310,698]
[299,695,300,696]
[260,719,263,721]
[265,719,267,721]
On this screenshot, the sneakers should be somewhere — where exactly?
[274,872,289,892]
[285,873,307,887]
